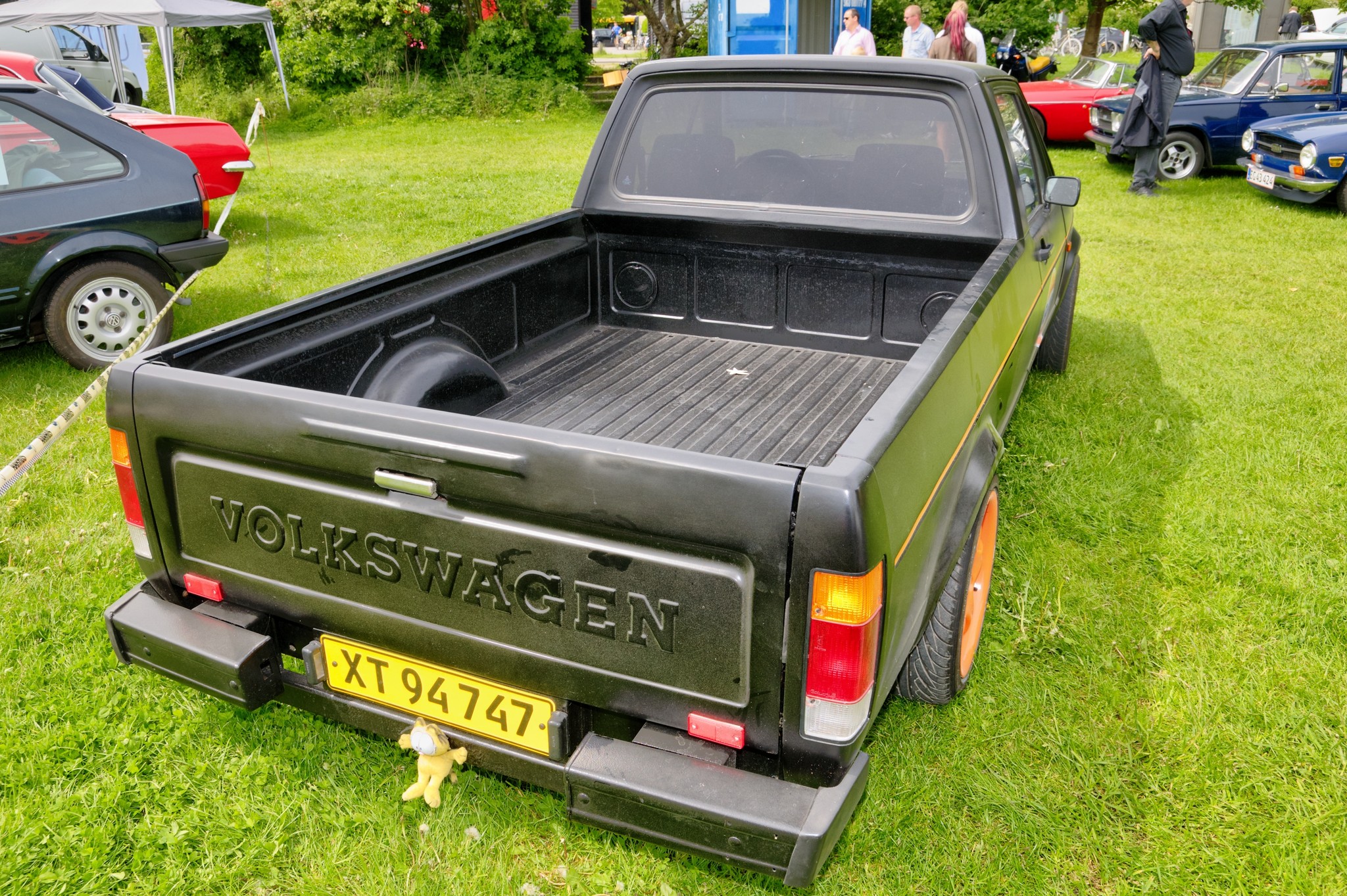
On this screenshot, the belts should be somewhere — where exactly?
[1160,68,1182,78]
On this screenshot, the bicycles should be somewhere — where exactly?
[615,47,662,91]
[1074,26,1118,57]
[1040,26,1082,58]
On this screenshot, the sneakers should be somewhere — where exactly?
[1127,180,1161,198]
[1146,182,1170,191]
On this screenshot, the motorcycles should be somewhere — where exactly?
[990,29,1061,83]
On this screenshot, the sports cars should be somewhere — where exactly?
[1012,58,1144,144]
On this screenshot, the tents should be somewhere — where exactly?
[0,0,292,118]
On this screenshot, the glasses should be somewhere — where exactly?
[903,15,915,19]
[844,17,849,20]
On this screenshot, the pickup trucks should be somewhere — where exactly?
[106,60,1080,891]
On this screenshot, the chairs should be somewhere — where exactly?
[644,133,734,201]
[835,143,947,215]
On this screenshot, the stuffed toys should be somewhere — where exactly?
[397,717,468,808]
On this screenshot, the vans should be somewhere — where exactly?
[0,16,151,108]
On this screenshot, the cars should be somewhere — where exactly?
[1295,8,1347,68]
[1087,40,1347,184]
[0,75,231,376]
[1072,27,1126,52]
[592,28,616,47]
[0,49,257,201]
[1236,111,1347,217]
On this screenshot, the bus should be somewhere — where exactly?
[596,14,651,46]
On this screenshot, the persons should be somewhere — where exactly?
[832,8,876,57]
[901,4,935,59]
[610,22,642,50]
[928,9,977,63]
[1277,6,1302,39]
[1106,0,1196,198]
[936,0,986,65]
